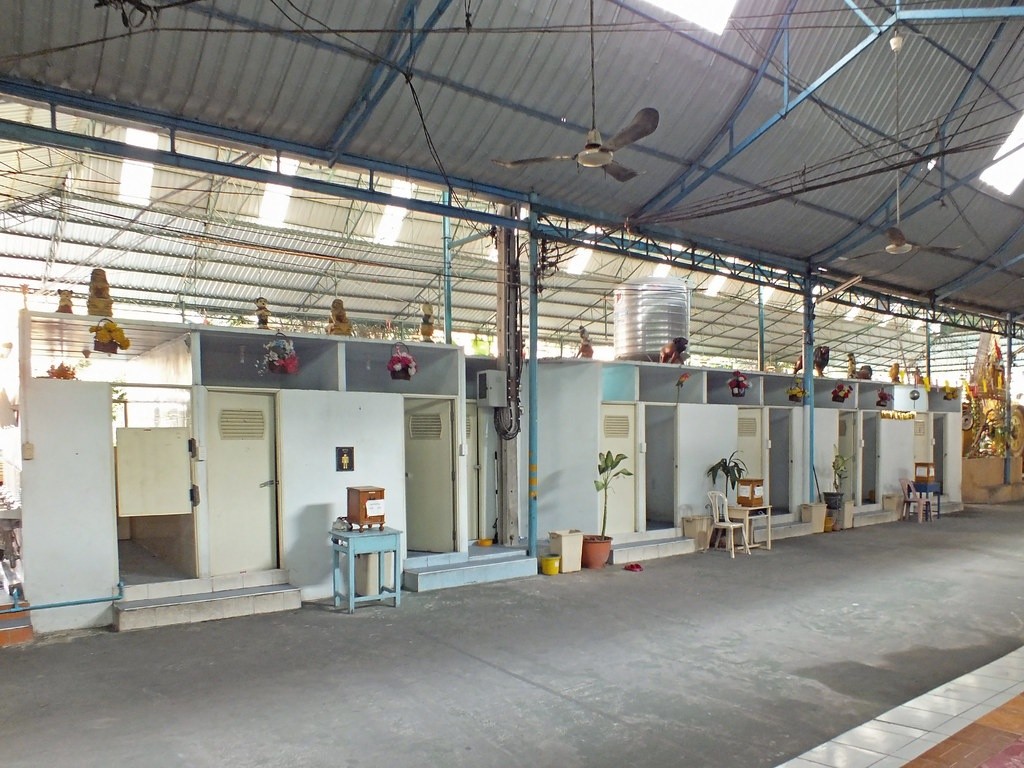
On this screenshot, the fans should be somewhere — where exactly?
[490,0,658,182]
[832,24,961,262]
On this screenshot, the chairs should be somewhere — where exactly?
[705,490,752,559]
[899,478,933,524]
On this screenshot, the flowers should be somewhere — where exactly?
[89,322,130,350]
[877,389,894,402]
[786,378,809,398]
[254,339,300,376]
[727,371,750,389]
[831,383,853,398]
[386,345,417,377]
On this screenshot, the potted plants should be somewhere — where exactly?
[822,444,854,510]
[581,450,633,569]
[705,450,749,548]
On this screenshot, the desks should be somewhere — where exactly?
[328,525,403,615]
[906,482,940,522]
[727,505,773,555]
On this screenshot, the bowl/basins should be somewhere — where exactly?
[479,539,492,546]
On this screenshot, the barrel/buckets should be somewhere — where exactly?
[825,515,835,532]
[541,554,561,575]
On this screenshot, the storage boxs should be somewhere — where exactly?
[346,486,386,533]
[915,463,936,483]
[736,478,764,507]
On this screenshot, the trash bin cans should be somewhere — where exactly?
[883,492,902,520]
[801,503,827,534]
[838,500,854,529]
[357,553,393,597]
[548,529,584,574]
[684,515,712,550]
